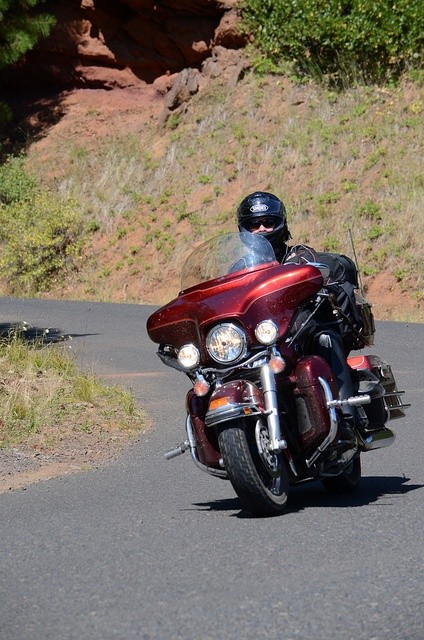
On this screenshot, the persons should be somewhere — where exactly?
[226,191,359,465]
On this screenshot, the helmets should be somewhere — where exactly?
[237,193,288,253]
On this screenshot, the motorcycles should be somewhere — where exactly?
[147,231,411,515]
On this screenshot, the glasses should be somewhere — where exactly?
[242,218,280,229]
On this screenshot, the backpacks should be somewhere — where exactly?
[289,245,376,350]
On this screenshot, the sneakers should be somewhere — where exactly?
[325,431,359,470]
